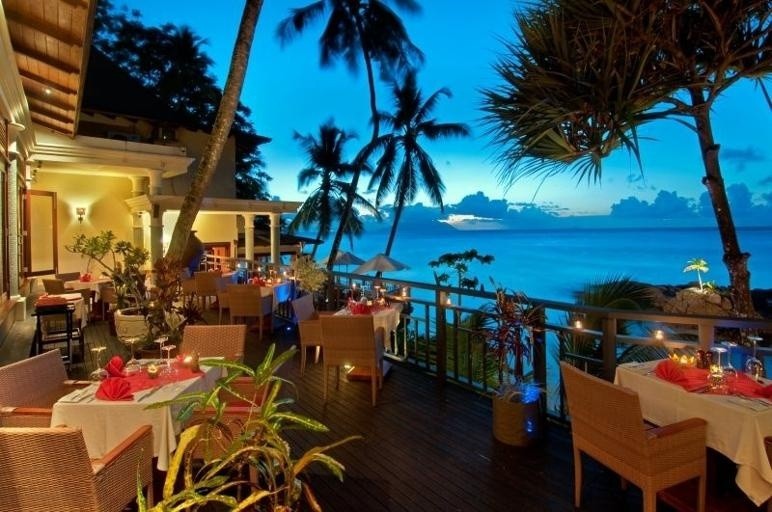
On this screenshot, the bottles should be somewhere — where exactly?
[190,349,199,372]
[696,349,713,369]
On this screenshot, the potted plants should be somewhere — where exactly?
[64,230,177,346]
[134,343,363,511]
[467,275,553,448]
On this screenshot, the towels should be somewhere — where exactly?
[751,382,772,398]
[654,358,710,395]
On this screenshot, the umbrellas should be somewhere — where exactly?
[323,251,368,265]
[350,252,412,278]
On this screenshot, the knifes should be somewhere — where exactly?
[69,386,98,404]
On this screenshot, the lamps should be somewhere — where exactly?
[75,206,87,218]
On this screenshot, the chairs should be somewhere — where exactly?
[101,287,118,321]
[177,326,246,375]
[0,424,153,510]
[319,315,384,412]
[183,277,210,311]
[194,271,221,311]
[292,293,338,379]
[217,278,238,327]
[556,361,708,510]
[227,284,272,341]
[0,347,93,428]
[178,376,269,509]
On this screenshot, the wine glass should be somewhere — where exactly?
[347,281,391,314]
[707,334,767,395]
[91,336,180,392]
[239,266,278,285]
